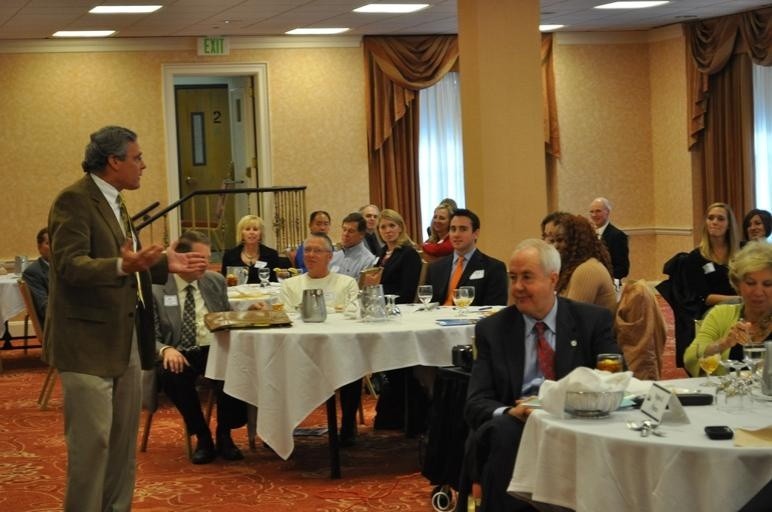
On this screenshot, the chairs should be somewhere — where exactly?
[412,258,428,303]
[616,279,662,384]
[16,277,59,411]
[358,267,385,290]
[139,371,255,459]
[181,178,234,252]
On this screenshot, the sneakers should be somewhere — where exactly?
[340,421,358,448]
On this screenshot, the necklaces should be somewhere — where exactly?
[240,248,259,266]
[740,314,772,341]
[384,248,394,257]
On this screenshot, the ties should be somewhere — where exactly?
[179,284,197,351]
[115,194,142,310]
[443,256,465,305]
[534,322,558,381]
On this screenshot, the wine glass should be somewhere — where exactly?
[715,360,743,416]
[384,295,402,320]
[697,343,722,387]
[417,285,433,313]
[276,269,290,283]
[742,343,766,370]
[291,269,303,277]
[238,267,250,287]
[258,268,270,290]
[459,286,475,313]
[725,363,753,415]
[741,359,769,410]
[452,289,468,318]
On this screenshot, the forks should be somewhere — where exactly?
[625,421,668,436]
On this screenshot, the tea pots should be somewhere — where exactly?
[302,288,327,322]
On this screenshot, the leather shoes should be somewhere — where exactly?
[216,432,244,461]
[191,435,216,464]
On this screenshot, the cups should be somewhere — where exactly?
[271,295,284,310]
[226,266,242,286]
[15,255,28,275]
[323,291,335,314]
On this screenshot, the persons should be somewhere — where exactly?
[454,236,629,512]
[21,226,51,331]
[682,240,771,377]
[421,202,454,263]
[741,207,772,247]
[359,203,386,259]
[421,208,509,432]
[540,212,561,244]
[327,212,376,291]
[373,209,423,431]
[38,124,212,512]
[151,230,248,466]
[222,213,280,287]
[685,201,746,320]
[280,233,364,449]
[548,212,620,321]
[294,210,334,273]
[588,195,631,288]
[427,198,459,236]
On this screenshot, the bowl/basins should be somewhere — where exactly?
[564,391,625,419]
